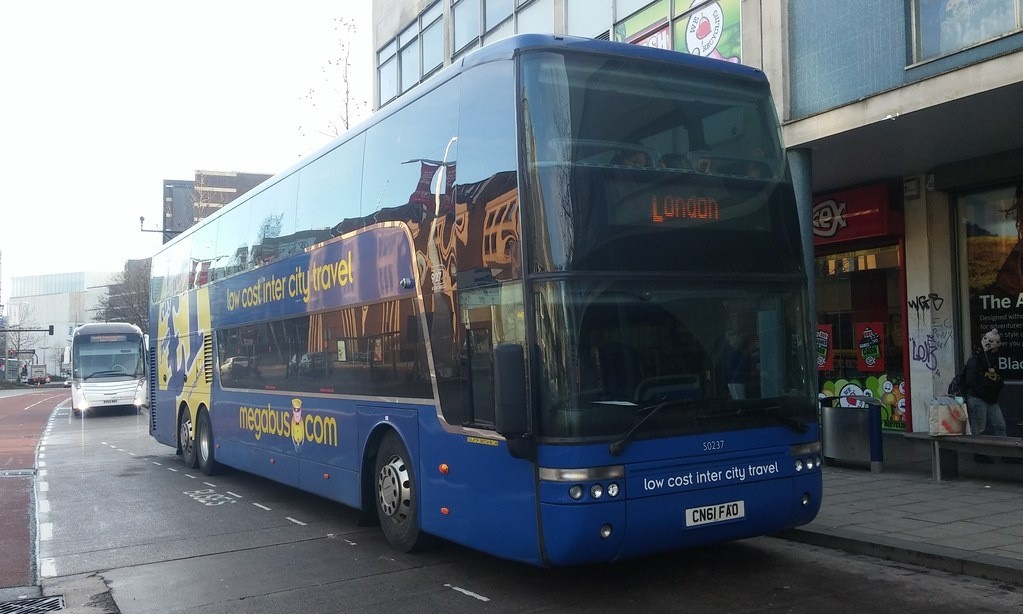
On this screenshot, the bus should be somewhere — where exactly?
[64,321,151,416]
[147,33,823,574]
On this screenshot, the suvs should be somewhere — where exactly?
[456,344,495,376]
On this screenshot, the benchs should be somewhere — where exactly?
[903,431,1023,480]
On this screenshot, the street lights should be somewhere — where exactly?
[39,347,50,364]
[5,302,29,382]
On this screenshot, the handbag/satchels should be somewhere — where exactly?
[928,394,969,436]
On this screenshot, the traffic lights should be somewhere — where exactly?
[22,365,24,370]
[10,350,13,355]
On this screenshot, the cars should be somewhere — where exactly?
[290,352,302,374]
[218,356,249,377]
[45,376,50,383]
[64,376,73,388]
[299,353,333,370]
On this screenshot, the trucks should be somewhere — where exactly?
[28,364,46,384]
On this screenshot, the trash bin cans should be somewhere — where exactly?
[821,395,883,473]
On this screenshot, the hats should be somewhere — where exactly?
[980,328,1002,372]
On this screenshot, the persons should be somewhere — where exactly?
[963,329,1012,467]
